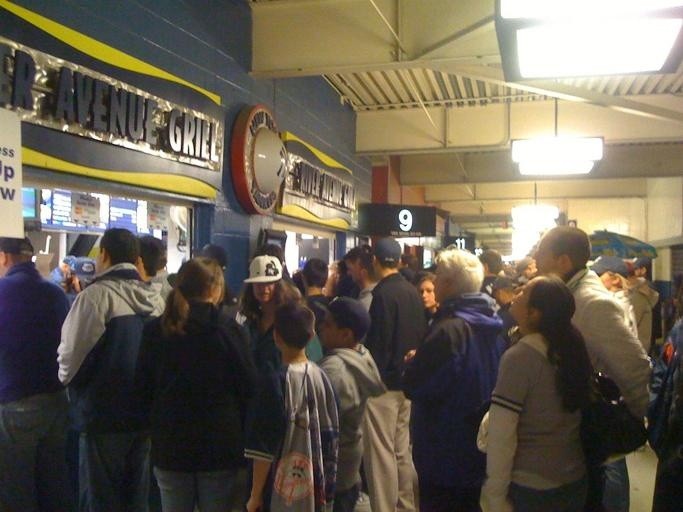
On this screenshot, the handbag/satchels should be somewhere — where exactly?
[589,368,647,457]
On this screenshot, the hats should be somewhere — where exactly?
[71,257,95,275]
[0,236,33,255]
[589,255,627,278]
[243,254,283,284]
[368,238,401,258]
[193,243,228,270]
[632,256,649,268]
[65,255,75,265]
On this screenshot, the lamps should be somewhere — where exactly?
[513,205,559,231]
[511,137,602,177]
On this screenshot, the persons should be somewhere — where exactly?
[311,296,386,512]
[0,236,72,512]
[343,244,377,313]
[239,254,321,373]
[137,233,165,283]
[59,258,95,309]
[401,253,418,277]
[479,249,509,310]
[143,257,250,512]
[633,256,651,282]
[400,247,511,512]
[244,302,340,512]
[589,255,638,341]
[301,256,329,330]
[532,224,652,423]
[645,318,683,512]
[56,227,166,512]
[515,256,536,286]
[416,274,437,320]
[477,274,596,512]
[361,236,416,512]
